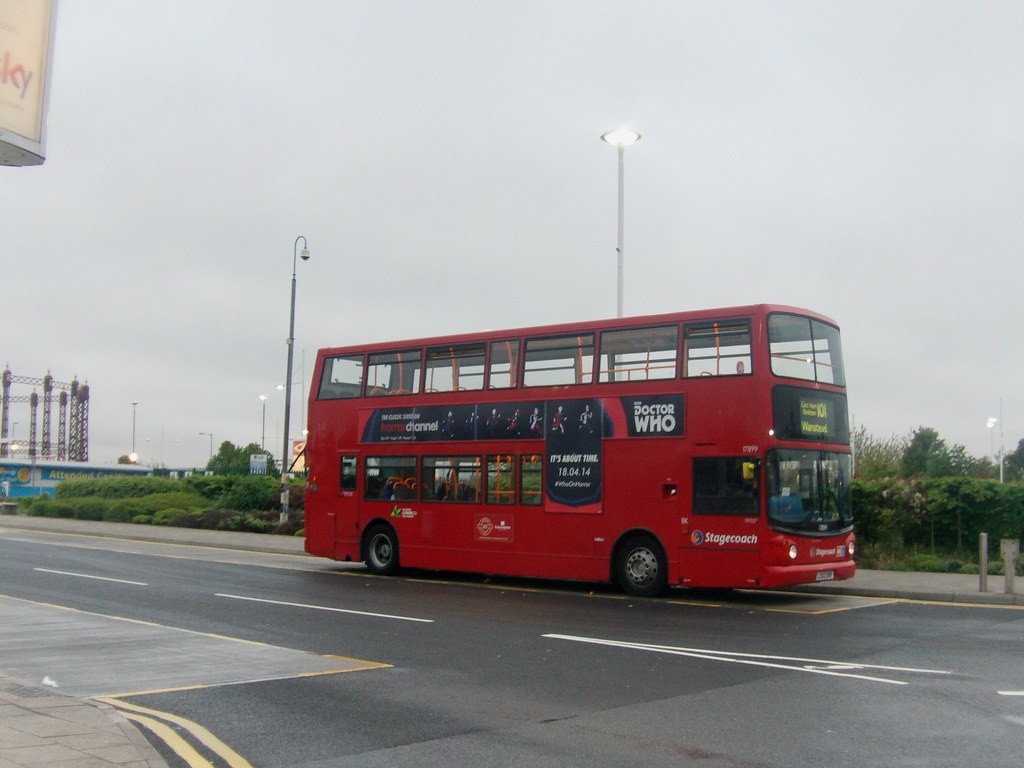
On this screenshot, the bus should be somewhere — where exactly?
[303,303,856,597]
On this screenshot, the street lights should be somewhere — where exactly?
[129,401,139,453]
[198,432,213,460]
[986,417,997,455]
[279,235,310,525]
[11,421,18,459]
[275,380,303,391]
[259,394,268,450]
[599,126,642,318]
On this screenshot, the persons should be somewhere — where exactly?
[440,404,595,437]
[732,478,758,515]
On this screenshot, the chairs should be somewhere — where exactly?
[317,381,528,399]
[381,476,485,502]
[700,371,712,376]
